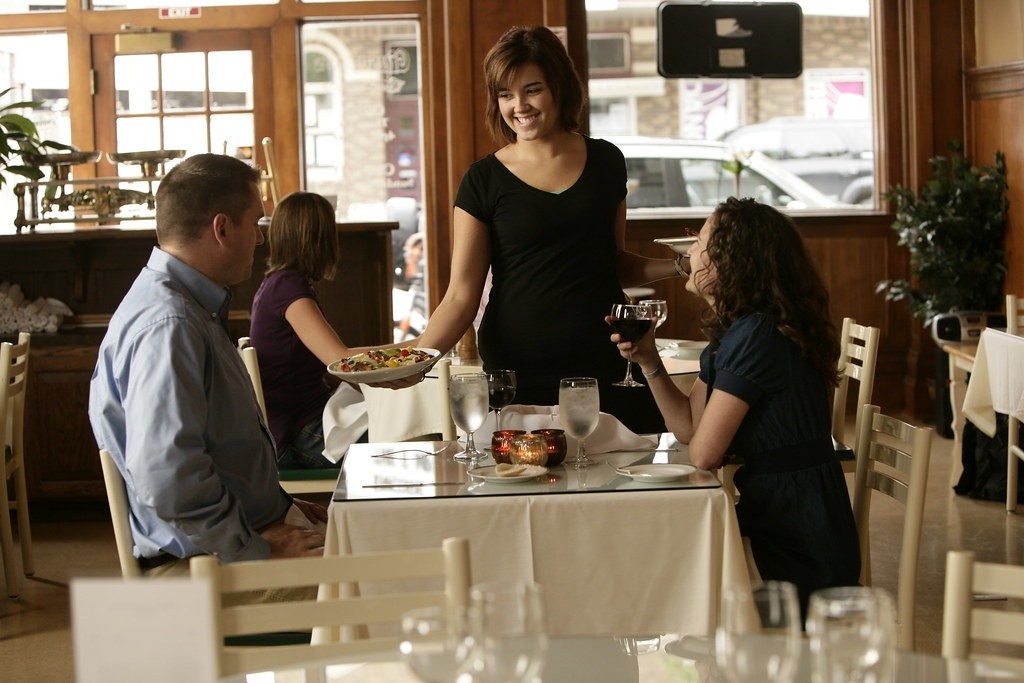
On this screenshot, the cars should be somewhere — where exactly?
[597,137,838,209]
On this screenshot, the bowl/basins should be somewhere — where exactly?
[653,236,698,257]
[669,341,710,359]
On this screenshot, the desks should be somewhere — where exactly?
[310,441,764,646]
[943,342,979,439]
[203,634,1024,683]
[404,338,708,435]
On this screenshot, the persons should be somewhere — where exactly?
[367,25,691,435]
[399,234,423,340]
[88,153,329,636]
[251,193,422,470]
[605,196,862,632]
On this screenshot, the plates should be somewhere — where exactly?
[467,464,551,484]
[327,347,441,383]
[616,463,697,483]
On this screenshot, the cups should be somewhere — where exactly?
[714,579,802,683]
[397,578,547,683]
[806,587,897,682]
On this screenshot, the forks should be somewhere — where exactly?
[370,443,452,458]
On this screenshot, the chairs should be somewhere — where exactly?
[0,331,35,598]
[1006,295,1024,512]
[190,537,470,677]
[799,404,933,651]
[239,337,340,493]
[942,551,1024,670]
[98,448,142,576]
[725,317,880,586]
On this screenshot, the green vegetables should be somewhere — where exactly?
[371,350,384,369]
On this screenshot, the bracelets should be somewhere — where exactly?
[641,359,664,378]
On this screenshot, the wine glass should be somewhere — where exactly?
[637,299,668,358]
[559,377,600,466]
[610,302,652,387]
[483,369,517,451]
[449,373,489,460]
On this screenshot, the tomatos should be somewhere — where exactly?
[385,346,412,367]
[342,364,350,372]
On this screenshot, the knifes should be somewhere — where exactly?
[362,481,466,488]
[607,449,680,453]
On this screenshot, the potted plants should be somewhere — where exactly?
[873,138,1012,439]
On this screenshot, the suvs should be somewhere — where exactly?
[682,115,872,206]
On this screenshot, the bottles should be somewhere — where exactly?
[491,429,567,468]
